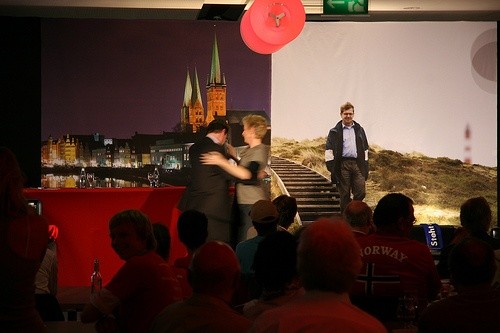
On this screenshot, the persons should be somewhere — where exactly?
[198,115,271,246]
[324,102,368,216]
[0,144,500,333]
[177,119,265,248]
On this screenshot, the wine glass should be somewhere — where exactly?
[87,173,95,190]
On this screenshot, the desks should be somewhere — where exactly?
[23,184,189,286]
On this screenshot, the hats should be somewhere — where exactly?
[248,200,280,224]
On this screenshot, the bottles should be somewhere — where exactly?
[147,170,159,188]
[79,168,87,189]
[89,257,103,302]
